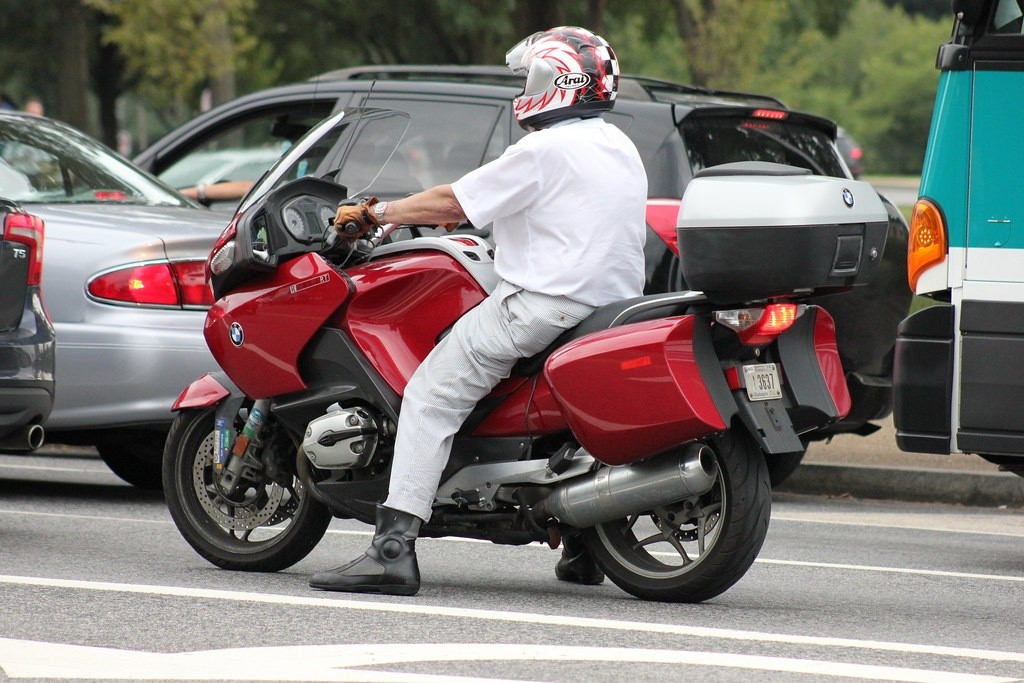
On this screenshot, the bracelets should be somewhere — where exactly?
[198,183,206,199]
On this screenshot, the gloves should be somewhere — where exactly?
[335,196,378,240]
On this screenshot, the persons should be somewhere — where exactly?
[307,26,649,596]
[179,180,256,198]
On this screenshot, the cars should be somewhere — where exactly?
[0,106,236,498]
[0,198,59,460]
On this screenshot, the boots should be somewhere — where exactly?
[308,503,422,595]
[554,536,606,585]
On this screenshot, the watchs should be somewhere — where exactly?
[374,201,388,226]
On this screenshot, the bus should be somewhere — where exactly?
[891,0,1024,481]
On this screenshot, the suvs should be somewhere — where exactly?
[124,63,917,495]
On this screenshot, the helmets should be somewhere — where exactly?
[270,105,344,162]
[511,26,621,129]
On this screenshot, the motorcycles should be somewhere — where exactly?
[159,105,890,604]
